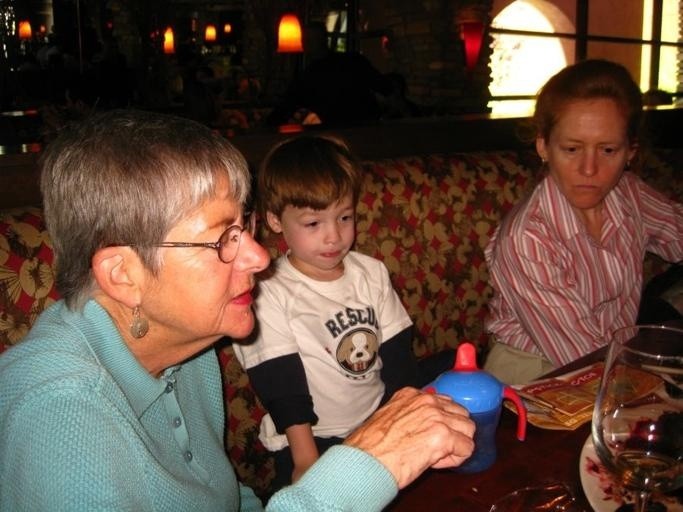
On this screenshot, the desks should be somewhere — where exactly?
[374,324,683,512]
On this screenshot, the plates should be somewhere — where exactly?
[577,398,682,511]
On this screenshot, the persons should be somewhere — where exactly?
[43,83,99,127]
[232,133,457,493]
[0,109,477,512]
[482,58,683,390]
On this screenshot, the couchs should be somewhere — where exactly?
[0,151,683,483]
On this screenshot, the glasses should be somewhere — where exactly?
[106,212,256,263]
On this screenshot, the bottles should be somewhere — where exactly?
[417,341,529,478]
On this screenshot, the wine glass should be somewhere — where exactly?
[588,323,682,512]
